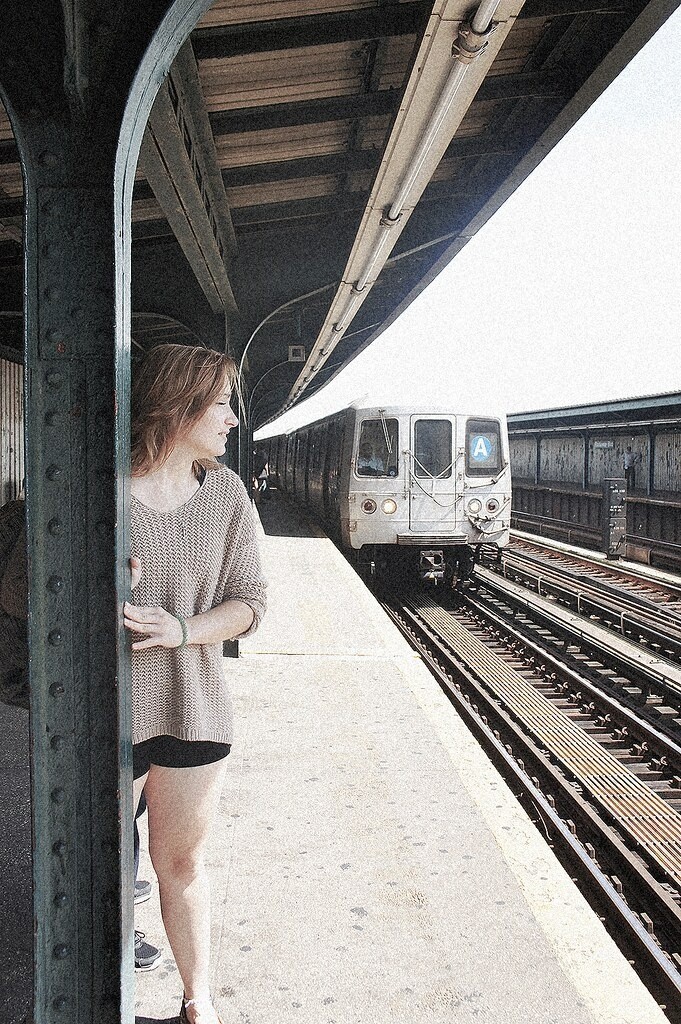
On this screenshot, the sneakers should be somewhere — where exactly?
[134,880,152,905]
[134,929,163,972]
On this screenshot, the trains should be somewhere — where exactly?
[253,404,513,600]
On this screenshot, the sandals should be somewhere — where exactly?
[179,989,224,1024]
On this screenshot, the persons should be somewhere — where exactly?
[357,443,384,472]
[0,346,267,1024]
[621,446,639,496]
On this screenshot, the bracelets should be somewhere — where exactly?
[175,614,188,653]
